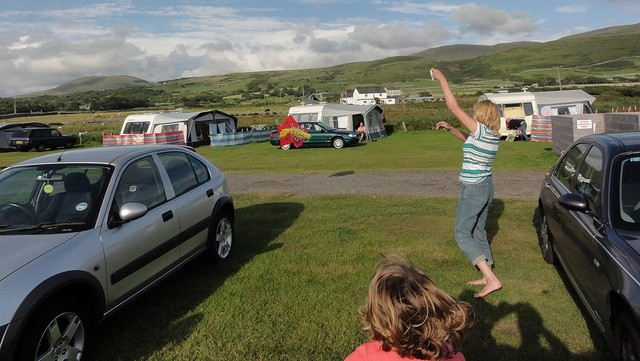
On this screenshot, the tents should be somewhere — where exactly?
[532,89,596,117]
[150,110,239,149]
[321,104,388,142]
[0,121,50,153]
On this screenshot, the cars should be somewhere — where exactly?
[270,121,361,151]
[539,132,640,360]
[6,127,80,151]
[1,144,235,361]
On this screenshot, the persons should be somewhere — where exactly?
[344,254,476,361]
[506,118,530,142]
[432,68,503,299]
[355,122,366,143]
